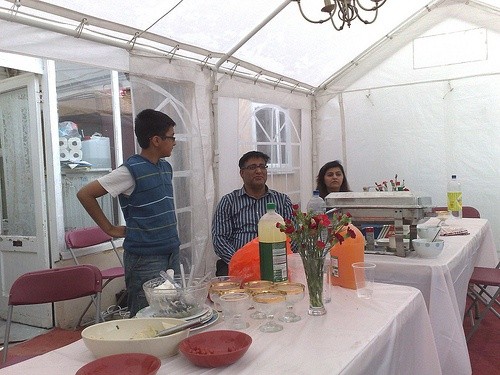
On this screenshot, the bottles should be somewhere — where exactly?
[328,216,365,290]
[307,190,326,244]
[366,227,375,251]
[447,175,462,218]
[258,203,288,282]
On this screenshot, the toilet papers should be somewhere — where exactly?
[58,138,67,149]
[59,150,70,162]
[67,137,82,149]
[69,150,83,162]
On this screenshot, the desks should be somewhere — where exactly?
[0,273,443,375]
[287,217,500,375]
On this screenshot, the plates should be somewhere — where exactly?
[131,306,219,331]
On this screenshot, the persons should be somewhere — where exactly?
[316,160,350,221]
[77,108,182,317]
[211,151,297,277]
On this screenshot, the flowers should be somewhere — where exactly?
[276,204,356,306]
[375,174,409,191]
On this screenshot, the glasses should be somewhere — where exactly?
[158,136,176,142]
[240,165,268,171]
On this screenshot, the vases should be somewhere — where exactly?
[298,251,328,317]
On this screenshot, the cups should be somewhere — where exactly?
[352,262,376,299]
[418,197,432,218]
[323,250,331,303]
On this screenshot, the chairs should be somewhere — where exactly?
[2,264,103,367]
[65,226,125,330]
[466,260,500,342]
[432,207,481,218]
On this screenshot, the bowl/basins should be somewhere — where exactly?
[416,224,440,238]
[143,274,211,317]
[81,317,190,360]
[178,330,252,367]
[75,353,162,375]
[412,238,445,258]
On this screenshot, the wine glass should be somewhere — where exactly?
[209,275,305,332]
[435,210,450,226]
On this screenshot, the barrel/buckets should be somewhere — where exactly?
[81,136,111,168]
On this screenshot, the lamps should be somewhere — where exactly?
[291,0,387,31]
